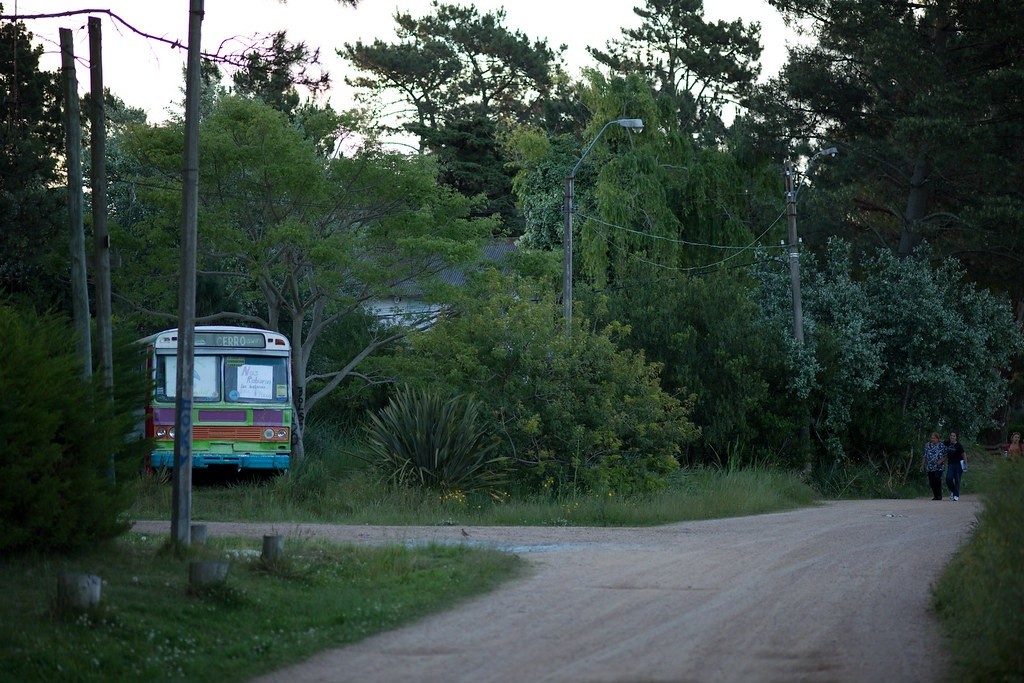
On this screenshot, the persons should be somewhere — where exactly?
[921,432,947,500]
[940,431,967,500]
[1006,432,1024,457]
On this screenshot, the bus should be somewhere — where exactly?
[113,325,292,484]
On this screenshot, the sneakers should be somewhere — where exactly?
[954,496,959,501]
[950,492,953,499]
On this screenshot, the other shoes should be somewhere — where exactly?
[931,496,941,500]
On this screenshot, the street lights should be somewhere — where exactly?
[563,118,645,334]
[783,146,839,486]
